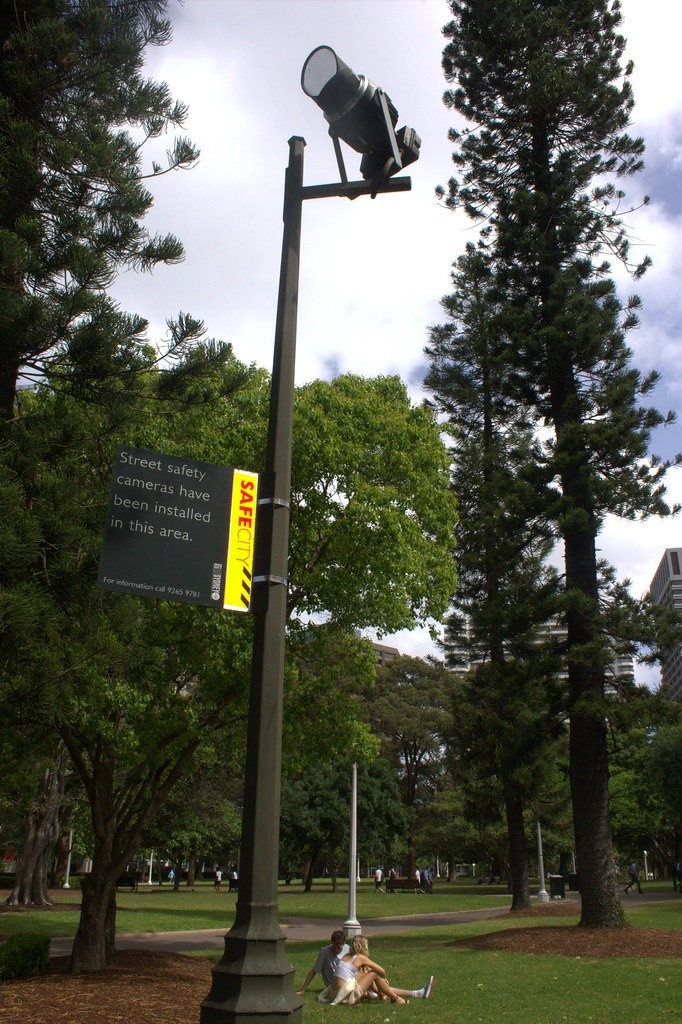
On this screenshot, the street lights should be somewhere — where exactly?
[643,850,649,881]
[197,44,419,1022]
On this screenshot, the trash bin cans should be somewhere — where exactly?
[550,875,565,898]
[569,873,579,892]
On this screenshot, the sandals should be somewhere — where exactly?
[391,996,407,1004]
[380,994,390,1000]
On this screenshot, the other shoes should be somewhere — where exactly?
[638,890,644,894]
[624,889,629,895]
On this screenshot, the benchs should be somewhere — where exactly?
[386,879,420,893]
[116,878,139,891]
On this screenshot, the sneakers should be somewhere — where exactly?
[422,976,434,999]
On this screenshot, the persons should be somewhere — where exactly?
[168,869,175,884]
[412,865,435,895]
[389,866,398,894]
[673,857,682,892]
[295,930,434,1000]
[623,858,645,893]
[374,865,386,894]
[213,867,238,893]
[329,935,411,1004]
